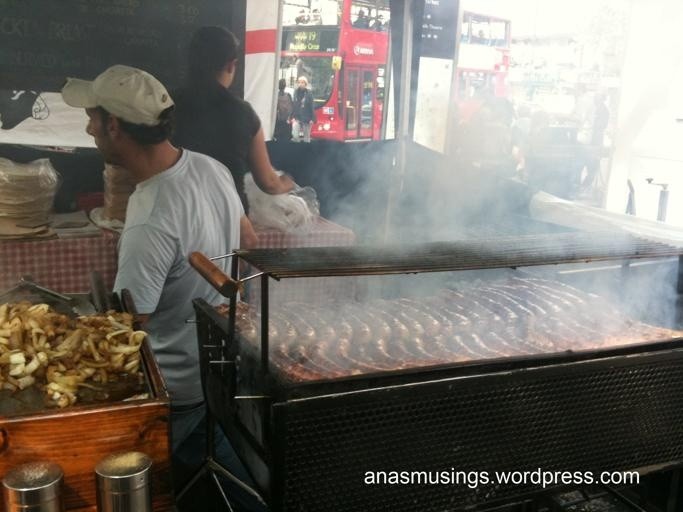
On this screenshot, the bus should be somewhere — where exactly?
[280,0,512,144]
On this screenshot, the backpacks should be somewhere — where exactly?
[277,93,292,119]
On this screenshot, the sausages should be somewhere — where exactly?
[215,276,673,385]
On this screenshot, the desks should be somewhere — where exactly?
[0,208,357,294]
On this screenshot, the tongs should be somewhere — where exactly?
[0,276,97,320]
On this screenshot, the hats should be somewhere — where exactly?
[61,64,175,127]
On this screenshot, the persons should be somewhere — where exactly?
[61,65,259,512]
[167,26,296,303]
[274,79,293,142]
[293,76,315,142]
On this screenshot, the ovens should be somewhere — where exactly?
[190,230,683,511]
